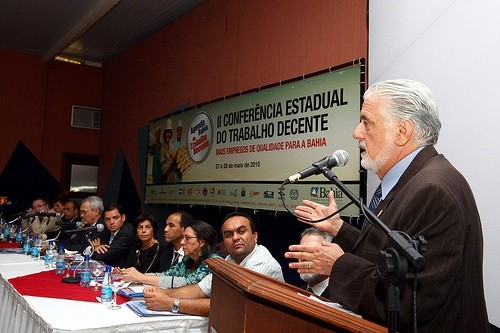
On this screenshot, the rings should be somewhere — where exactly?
[308,262,311,269]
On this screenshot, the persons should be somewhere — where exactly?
[285,78,489,333]
[20,196,333,316]
[158,120,181,184]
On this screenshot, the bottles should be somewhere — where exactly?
[79,254,92,287]
[100,264,113,307]
[55,246,65,274]
[0,218,54,268]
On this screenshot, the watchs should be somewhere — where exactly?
[172,298,180,314]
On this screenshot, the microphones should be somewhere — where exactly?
[65,224,105,236]
[284,149,349,186]
[38,212,60,217]
[23,210,46,220]
[13,208,33,218]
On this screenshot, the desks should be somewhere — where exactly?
[0,254,209,333]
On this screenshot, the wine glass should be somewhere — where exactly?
[108,272,125,310]
[64,255,73,278]
[90,260,105,292]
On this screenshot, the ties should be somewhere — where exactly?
[172,252,183,266]
[109,233,115,244]
[359,183,382,235]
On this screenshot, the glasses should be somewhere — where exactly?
[80,209,96,214]
[182,234,197,241]
